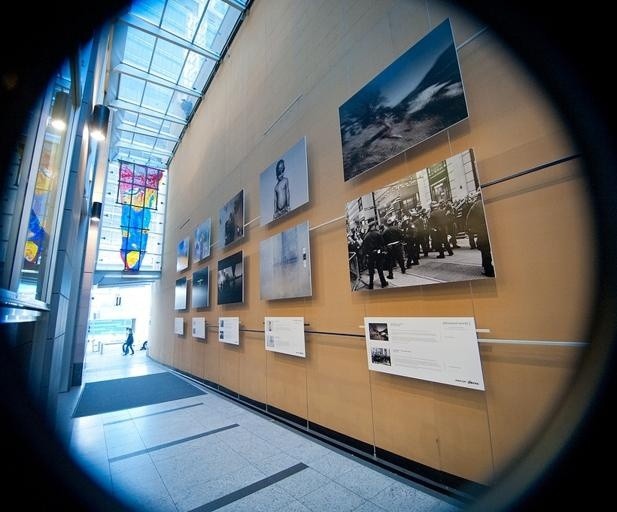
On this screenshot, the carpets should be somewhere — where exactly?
[70,372,207,418]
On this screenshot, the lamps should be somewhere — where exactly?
[87,202,102,221]
[85,104,110,141]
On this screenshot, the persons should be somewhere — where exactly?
[272,159,291,220]
[346,186,495,290]
[123,328,135,355]
[374,98,413,139]
[193,232,207,260]
